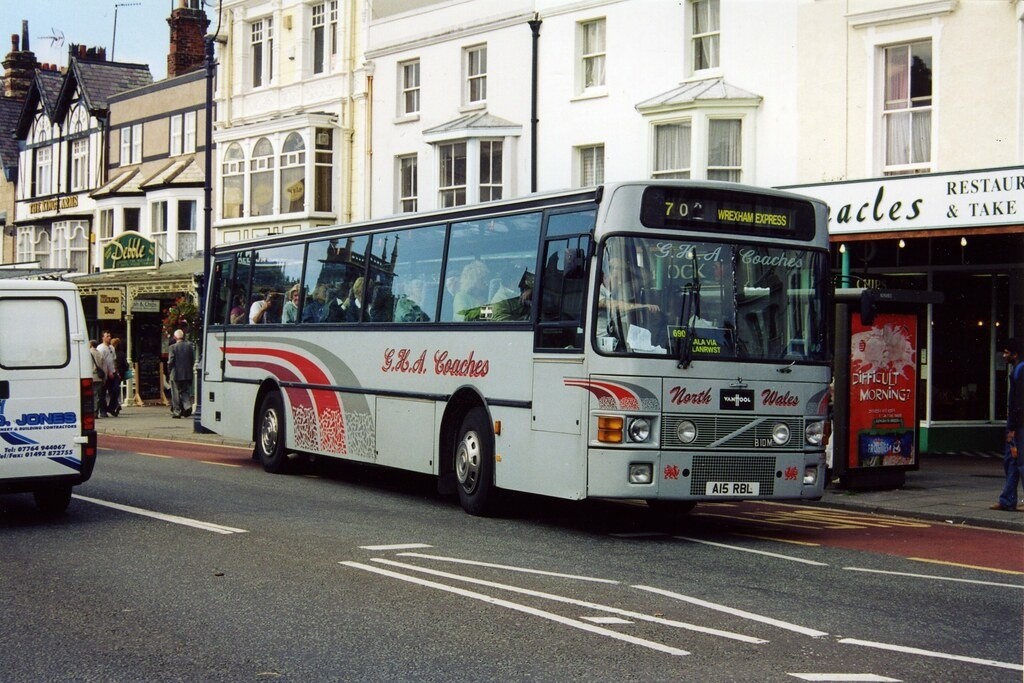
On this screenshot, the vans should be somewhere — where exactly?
[0,277,98,510]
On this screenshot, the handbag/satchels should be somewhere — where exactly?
[95,366,106,380]
[121,369,134,381]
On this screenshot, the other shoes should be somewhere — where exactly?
[989,501,1024,511]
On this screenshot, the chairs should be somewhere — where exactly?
[228,277,508,324]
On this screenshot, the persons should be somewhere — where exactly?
[491,264,534,322]
[392,277,424,322]
[596,257,665,354]
[96,329,120,420]
[439,270,461,320]
[231,293,247,324]
[108,337,130,418]
[89,338,108,421]
[825,376,842,486]
[166,329,196,418]
[328,279,355,322]
[989,336,1024,514]
[452,260,491,322]
[282,282,301,325]
[346,276,377,323]
[301,283,328,323]
[249,291,277,324]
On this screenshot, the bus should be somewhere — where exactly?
[196,178,830,517]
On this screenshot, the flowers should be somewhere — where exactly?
[161,297,200,342]
